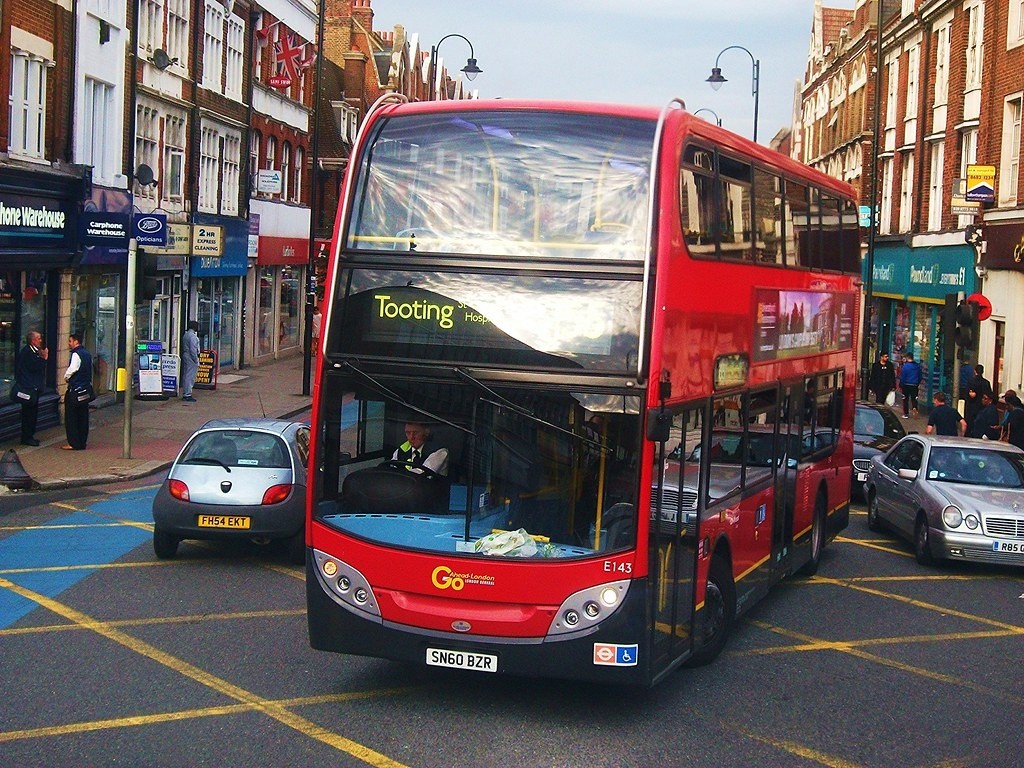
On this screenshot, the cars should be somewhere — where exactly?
[152,417,354,564]
[860,433,1024,572]
[850,397,920,499]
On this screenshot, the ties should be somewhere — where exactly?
[412,450,420,469]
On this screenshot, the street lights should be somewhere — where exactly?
[429,33,484,103]
[706,45,762,142]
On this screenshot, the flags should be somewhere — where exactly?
[275,34,300,80]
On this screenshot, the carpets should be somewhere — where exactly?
[212,374,250,384]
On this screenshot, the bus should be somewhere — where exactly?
[303,91,875,695]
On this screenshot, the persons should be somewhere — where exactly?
[15,331,48,446]
[391,417,449,480]
[62,334,93,449]
[925,391,967,436]
[870,352,896,404]
[930,453,960,478]
[311,306,322,356]
[183,321,200,401]
[985,459,1007,483]
[899,352,923,419]
[958,356,1024,450]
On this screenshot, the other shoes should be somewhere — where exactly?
[902,415,909,418]
[21,439,39,446]
[912,407,918,415]
[182,396,196,402]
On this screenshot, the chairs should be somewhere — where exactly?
[393,227,442,250]
[573,224,633,259]
[264,442,285,467]
[207,439,238,465]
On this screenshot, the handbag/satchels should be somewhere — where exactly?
[9,382,38,405]
[885,391,895,407]
[73,384,96,405]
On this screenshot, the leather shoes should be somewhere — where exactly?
[62,445,74,449]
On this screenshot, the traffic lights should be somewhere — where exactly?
[137,246,158,302]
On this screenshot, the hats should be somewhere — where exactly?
[1002,390,1016,398]
